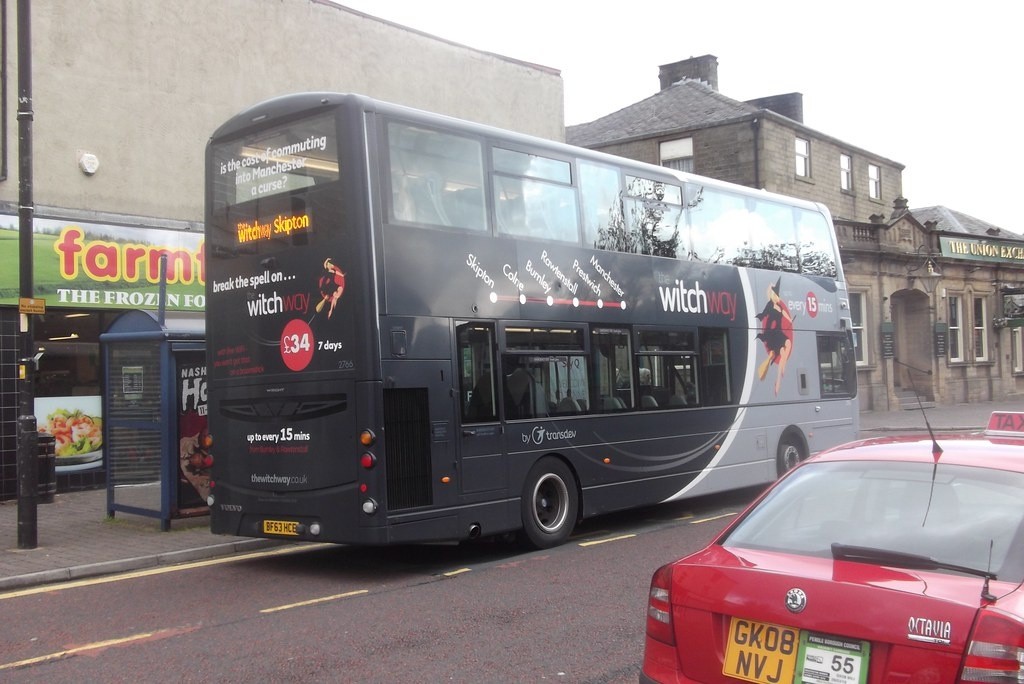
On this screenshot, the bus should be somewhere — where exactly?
[204,89,858,544]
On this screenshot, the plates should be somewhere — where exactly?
[54,446,104,467]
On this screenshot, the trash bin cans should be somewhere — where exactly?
[36,432,57,504]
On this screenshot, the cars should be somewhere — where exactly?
[640,411,1024,683]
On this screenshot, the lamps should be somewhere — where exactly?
[904,245,944,293]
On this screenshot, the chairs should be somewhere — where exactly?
[545,395,688,414]
[388,172,600,248]
